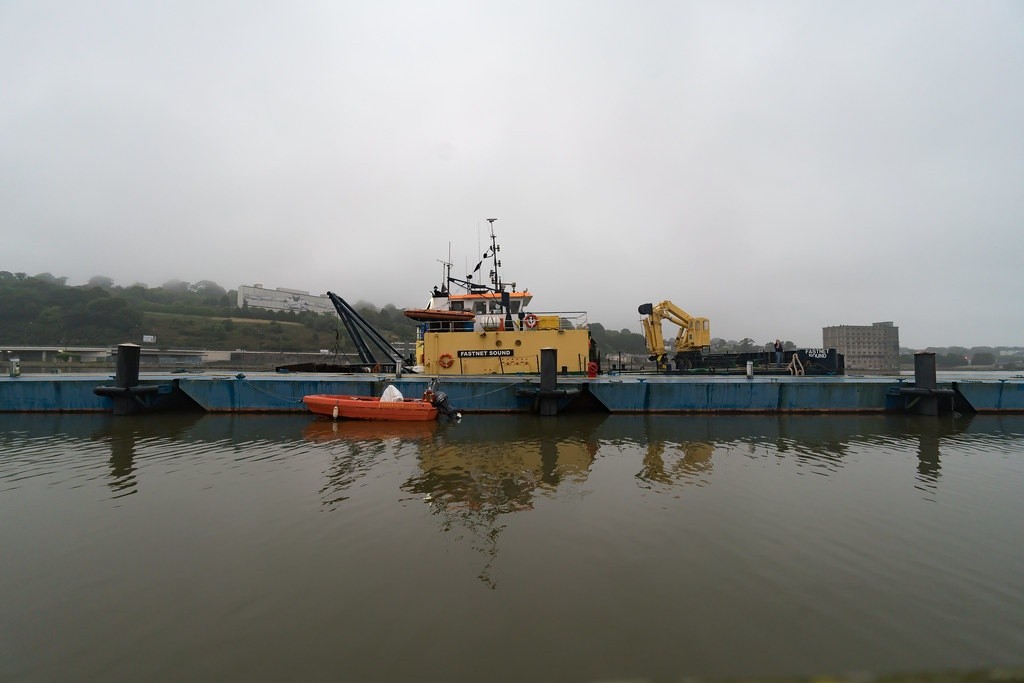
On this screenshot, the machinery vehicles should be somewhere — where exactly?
[638,300,711,375]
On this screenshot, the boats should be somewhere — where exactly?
[274,217,801,375]
[302,376,464,426]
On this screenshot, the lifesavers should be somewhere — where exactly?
[525,315,538,328]
[709,365,715,374]
[438,354,454,368]
